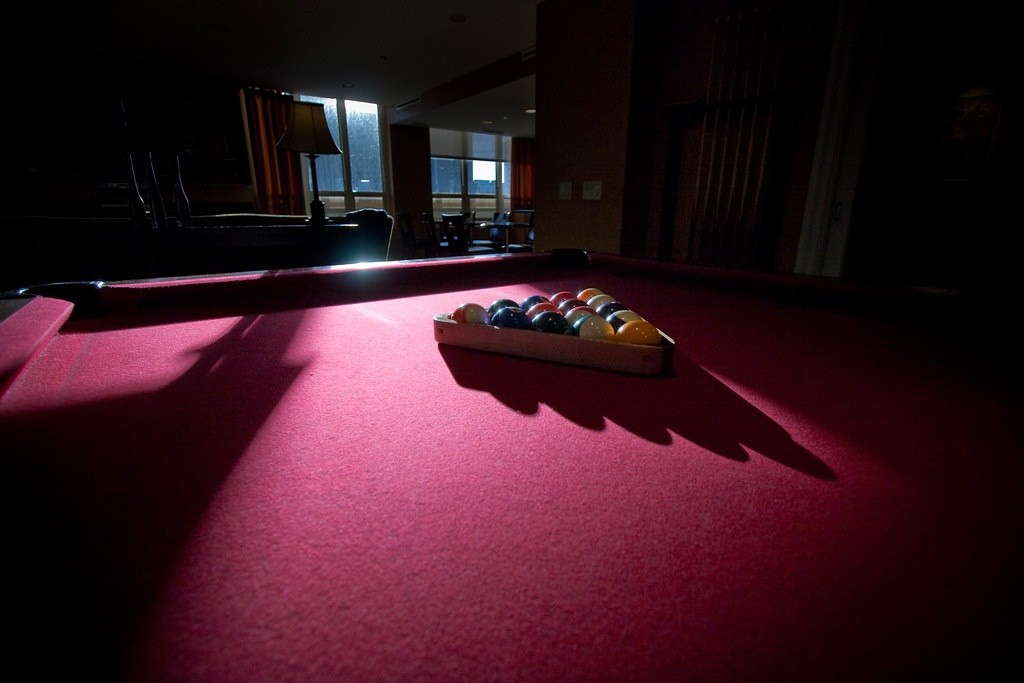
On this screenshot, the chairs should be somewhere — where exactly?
[394,210,494,255]
[500,228,534,253]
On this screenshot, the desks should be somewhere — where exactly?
[481,221,529,253]
[0,246,1024,683]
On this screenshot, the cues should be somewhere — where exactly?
[684,12,785,267]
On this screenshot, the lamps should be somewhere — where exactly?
[275,100,343,225]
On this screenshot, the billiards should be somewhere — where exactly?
[450,286,661,344]
